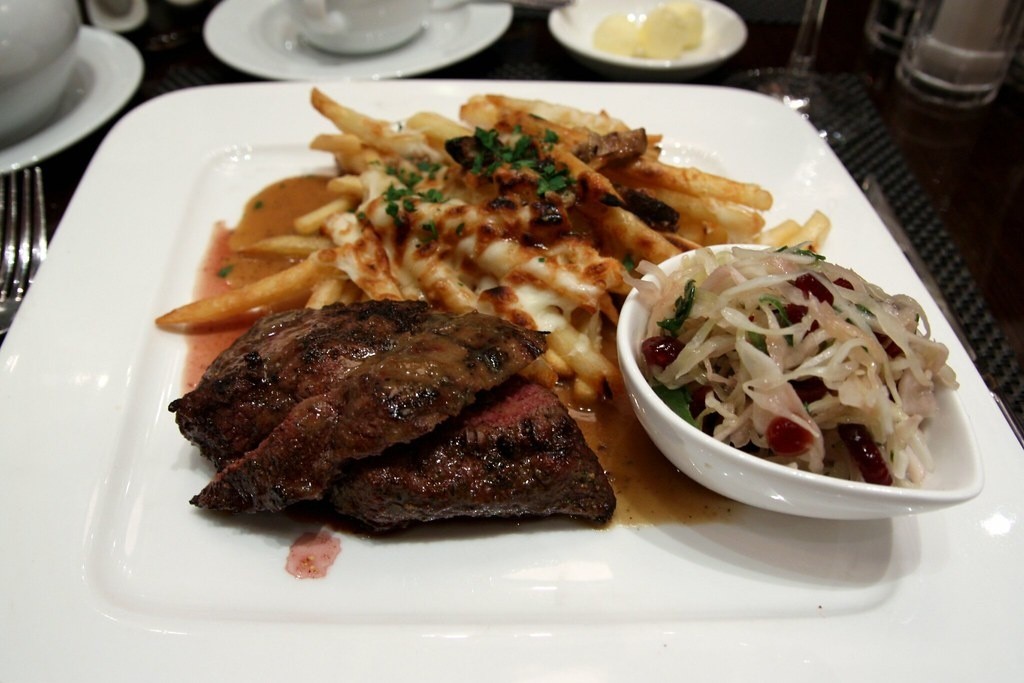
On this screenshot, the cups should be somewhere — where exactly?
[1,1,80,144]
[865,0,1023,110]
[282,0,428,56]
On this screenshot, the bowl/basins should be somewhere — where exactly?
[615,243,983,521]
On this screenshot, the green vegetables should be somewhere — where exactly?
[650,246,873,427]
[215,122,636,273]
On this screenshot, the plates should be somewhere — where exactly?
[549,0,748,83]
[0,82,1024,682]
[0,22,145,175]
[201,0,514,83]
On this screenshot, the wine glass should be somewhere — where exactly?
[725,0,881,143]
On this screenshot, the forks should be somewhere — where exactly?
[0,166,47,335]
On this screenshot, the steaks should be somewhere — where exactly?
[166,301,619,528]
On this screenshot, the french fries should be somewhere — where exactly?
[156,91,831,403]
